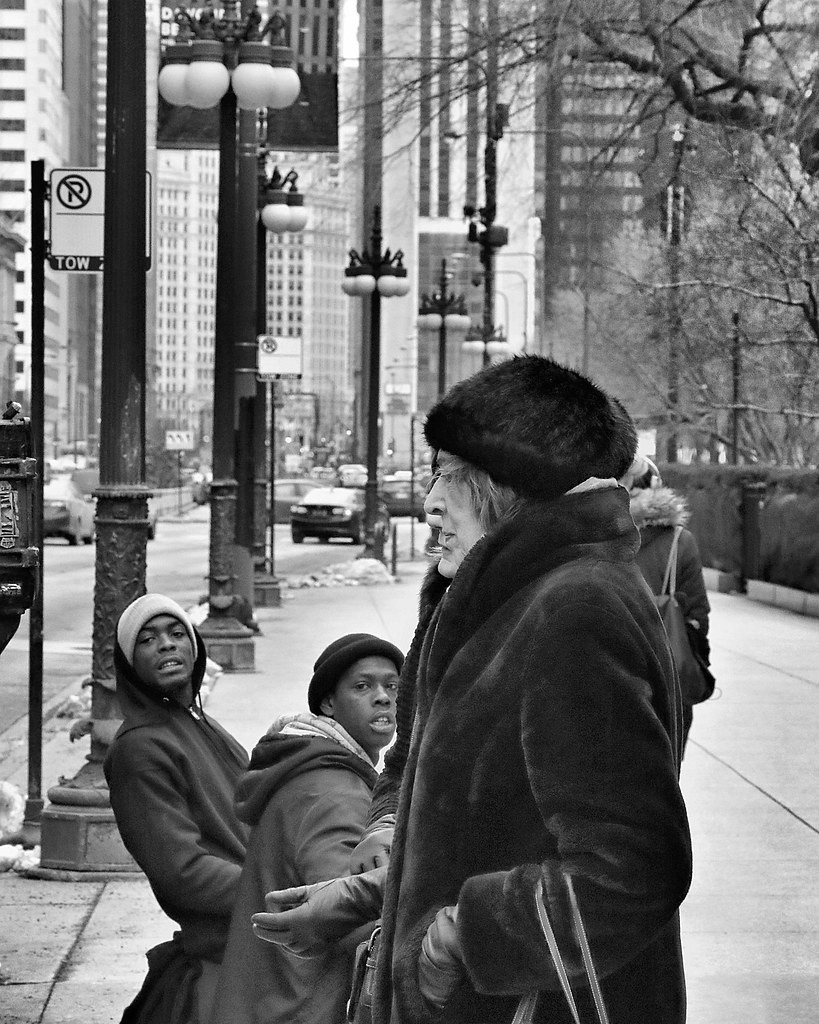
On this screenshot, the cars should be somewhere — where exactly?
[265,478,333,528]
[289,485,391,544]
[40,474,94,546]
[190,472,214,505]
[306,464,436,493]
[367,474,430,523]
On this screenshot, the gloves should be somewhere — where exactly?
[251,865,388,960]
[350,814,396,876]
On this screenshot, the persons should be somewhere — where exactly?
[246,439,492,1024]
[349,349,696,1023]
[223,637,409,1020]
[616,455,716,780]
[99,593,264,1020]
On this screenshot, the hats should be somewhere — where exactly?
[308,633,406,721]
[422,352,636,502]
[118,594,197,666]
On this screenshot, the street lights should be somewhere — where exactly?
[154,3,298,675]
[460,314,513,372]
[421,254,472,483]
[254,157,307,606]
[341,244,411,561]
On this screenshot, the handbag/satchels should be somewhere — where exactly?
[652,526,717,708]
